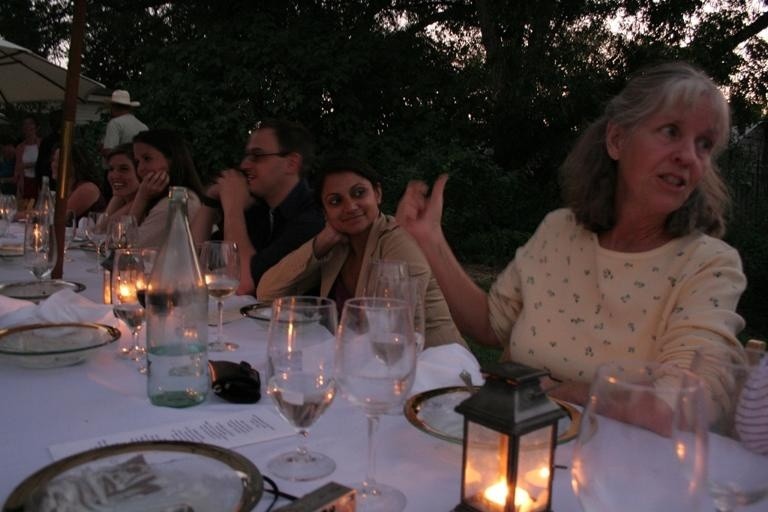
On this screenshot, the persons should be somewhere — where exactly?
[0,134,15,196]
[96,143,145,233]
[97,89,149,170]
[15,115,44,208]
[256,156,471,353]
[396,61,750,439]
[51,142,107,227]
[127,126,221,255]
[735,353,768,454]
[35,109,63,191]
[190,120,336,305]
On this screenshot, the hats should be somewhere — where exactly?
[104,88,141,110]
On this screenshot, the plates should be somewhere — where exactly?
[240,301,322,333]
[0,323,125,372]
[1,244,23,264]
[1,438,265,512]
[0,279,90,304]
[398,382,591,450]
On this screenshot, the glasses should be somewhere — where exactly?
[241,150,290,164]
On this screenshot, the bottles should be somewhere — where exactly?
[145,183,210,410]
[36,175,54,209]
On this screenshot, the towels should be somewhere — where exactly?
[0,288,111,328]
[416,339,485,397]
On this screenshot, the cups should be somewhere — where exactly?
[103,214,139,250]
[569,341,768,511]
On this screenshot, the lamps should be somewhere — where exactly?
[100,238,150,305]
[447,360,568,512]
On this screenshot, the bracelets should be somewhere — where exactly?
[203,196,220,208]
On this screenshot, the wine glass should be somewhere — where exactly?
[23,209,59,280]
[334,297,418,511]
[200,240,242,356]
[0,194,20,241]
[263,295,341,484]
[85,211,110,273]
[64,210,77,262]
[110,246,157,364]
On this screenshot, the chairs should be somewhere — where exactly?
[723,338,763,442]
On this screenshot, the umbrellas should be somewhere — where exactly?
[0,36,106,105]
